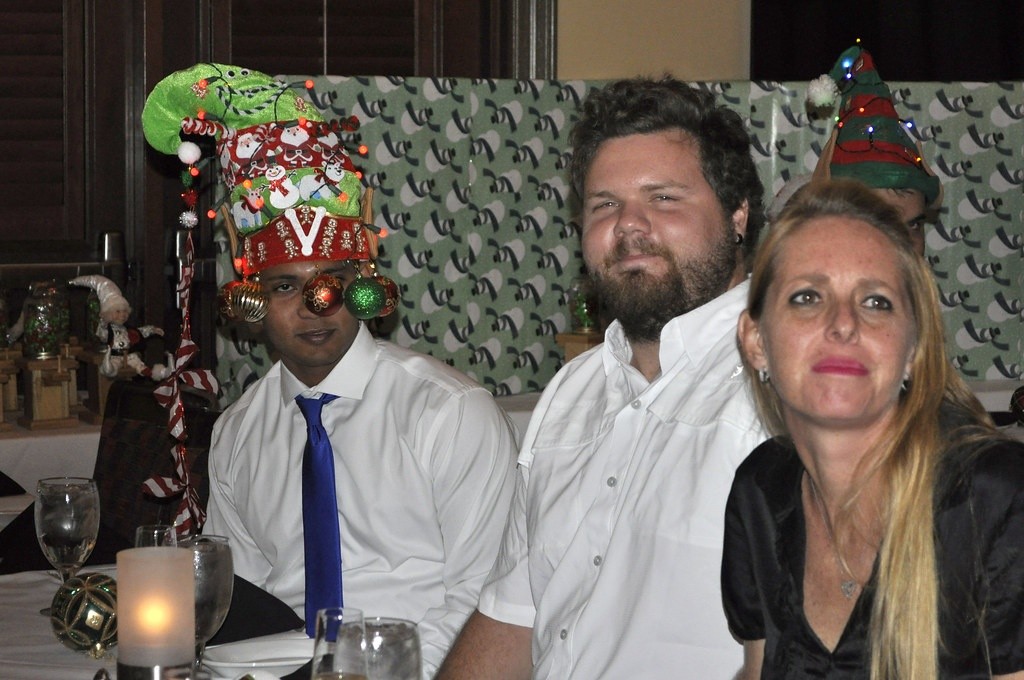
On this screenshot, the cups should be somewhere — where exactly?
[312,608,367,680]
[334,617,421,680]
[135,526,178,547]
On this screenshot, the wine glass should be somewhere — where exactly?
[34,476,100,615]
[178,535,233,680]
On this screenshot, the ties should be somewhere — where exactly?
[295,393,345,642]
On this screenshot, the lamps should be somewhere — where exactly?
[116,547,196,680]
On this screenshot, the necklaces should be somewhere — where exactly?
[807,476,877,598]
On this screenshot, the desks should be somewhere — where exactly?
[0,390,102,499]
[0,565,278,680]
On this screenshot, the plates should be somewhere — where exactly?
[200,634,314,680]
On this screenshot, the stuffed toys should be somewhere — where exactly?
[72,274,166,377]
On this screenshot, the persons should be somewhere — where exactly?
[142,63,518,680]
[720,177,1024,680]
[426,78,778,680]
[812,45,941,265]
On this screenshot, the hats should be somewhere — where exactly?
[808,37,942,205]
[140,61,400,324]
